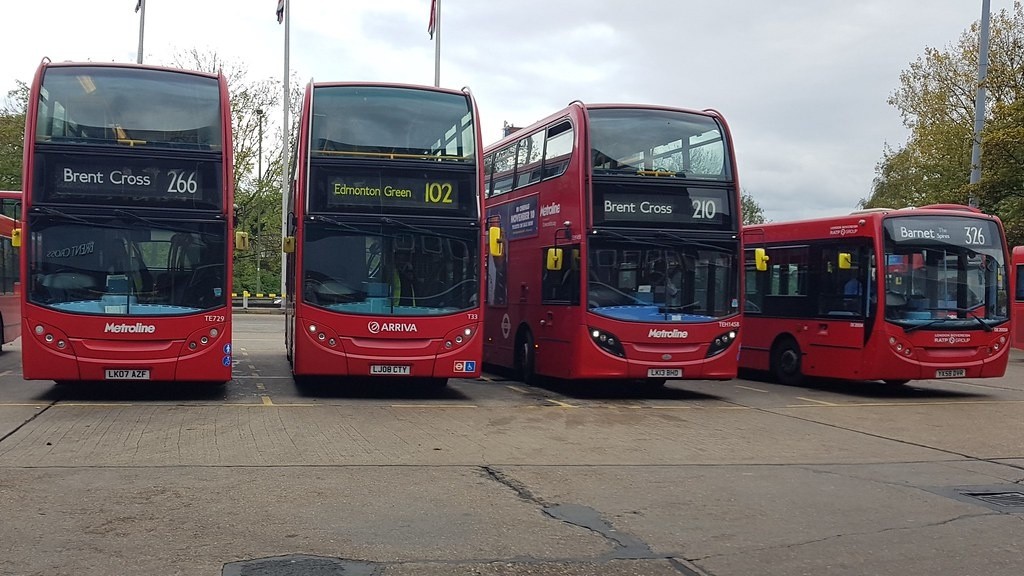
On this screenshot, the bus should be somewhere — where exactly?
[283,74,507,398]
[0,190,29,355]
[723,201,1011,407]
[438,98,744,393]
[867,246,1024,352]
[12,55,234,393]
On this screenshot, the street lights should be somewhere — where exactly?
[254,109,266,297]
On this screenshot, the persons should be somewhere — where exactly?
[106,239,143,304]
[386,251,416,308]
[639,259,678,306]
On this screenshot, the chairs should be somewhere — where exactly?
[317,139,461,161]
[596,167,695,178]
